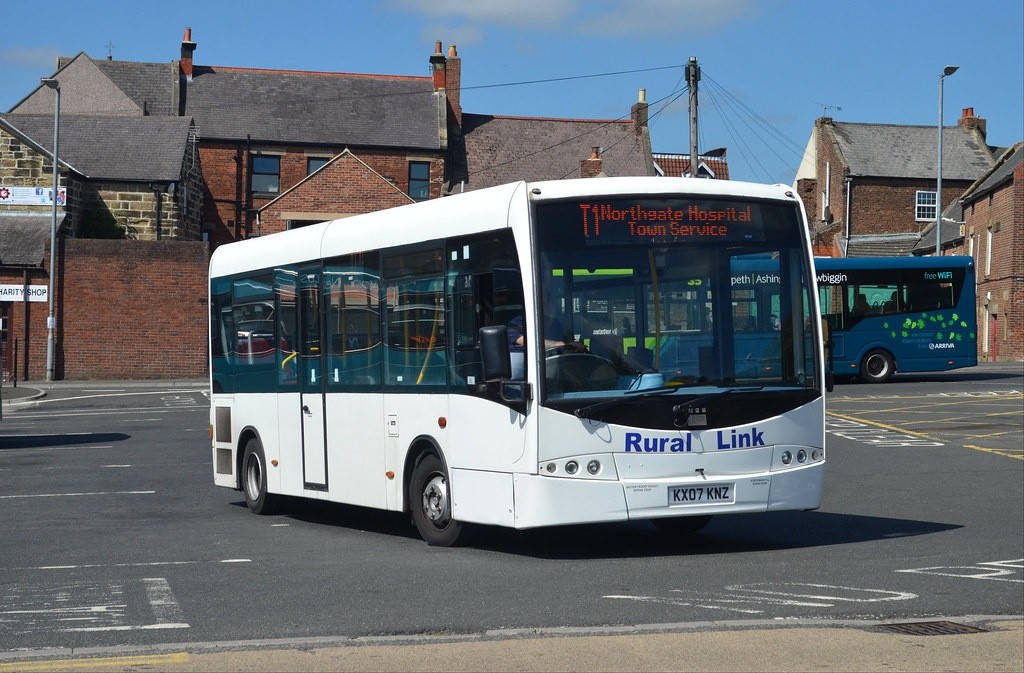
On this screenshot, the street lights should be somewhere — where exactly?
[41,77,61,381]
[934,64,960,256]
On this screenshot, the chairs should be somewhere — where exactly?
[622,317,635,337]
[872,298,916,312]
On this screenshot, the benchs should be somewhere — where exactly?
[237,328,345,383]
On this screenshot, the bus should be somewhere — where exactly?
[204,177,829,548]
[644,249,981,385]
[548,265,704,362]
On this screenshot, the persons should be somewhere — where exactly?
[507,288,588,382]
